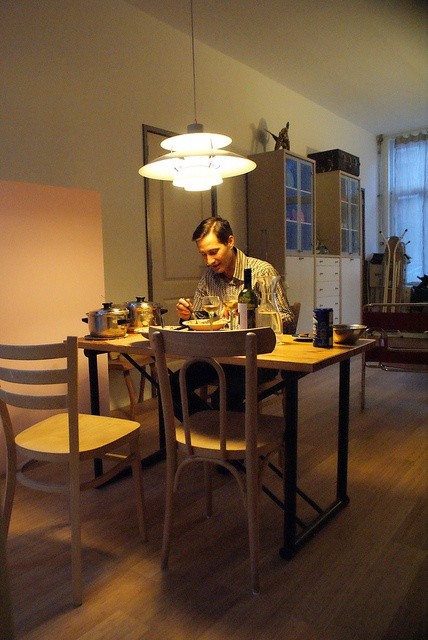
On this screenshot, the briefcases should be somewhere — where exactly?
[308,149,360,176]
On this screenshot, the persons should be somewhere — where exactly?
[174,216,296,334]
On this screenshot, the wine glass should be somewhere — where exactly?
[223,295,238,330]
[202,296,220,330]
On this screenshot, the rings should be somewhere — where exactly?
[176,303,180,308]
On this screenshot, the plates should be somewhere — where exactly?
[133,325,181,339]
[181,318,230,331]
[292,334,313,342]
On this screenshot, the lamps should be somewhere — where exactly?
[137,123,258,193]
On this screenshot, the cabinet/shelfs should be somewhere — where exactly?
[315,253,340,329]
[246,151,317,334]
[318,170,362,325]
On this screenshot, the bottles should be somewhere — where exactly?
[238,267,259,330]
[256,274,283,344]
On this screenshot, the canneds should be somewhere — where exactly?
[312,308,333,348]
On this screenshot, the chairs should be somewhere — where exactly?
[0,334,151,607]
[361,301,427,415]
[147,323,289,598]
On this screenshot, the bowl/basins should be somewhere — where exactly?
[333,324,368,344]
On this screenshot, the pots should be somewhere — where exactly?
[81,302,135,338]
[124,296,168,326]
